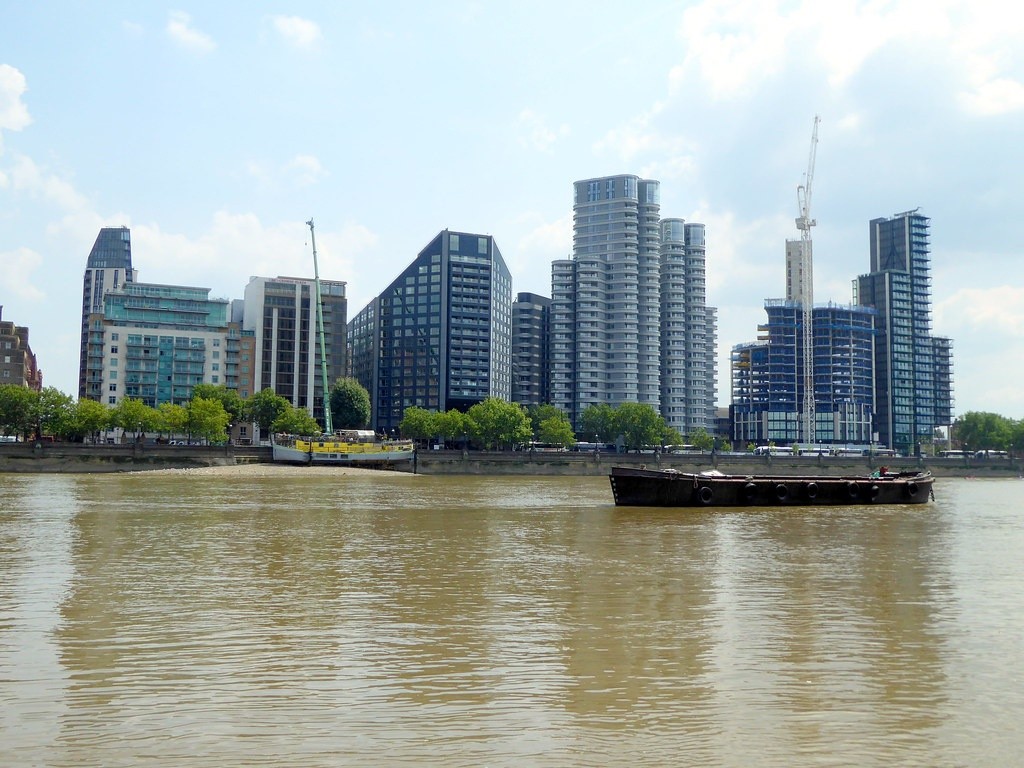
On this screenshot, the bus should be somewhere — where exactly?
[862,449,894,458]
[796,447,835,457]
[836,449,862,457]
[975,449,1008,460]
[618,444,662,454]
[520,441,569,453]
[663,444,703,455]
[937,449,974,459]
[752,445,794,457]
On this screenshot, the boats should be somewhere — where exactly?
[608,465,936,507]
[271,216,415,467]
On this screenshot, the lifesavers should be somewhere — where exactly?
[744,483,758,501]
[909,482,919,497]
[775,484,790,500]
[847,482,860,497]
[808,482,820,499]
[870,485,881,502]
[699,486,714,503]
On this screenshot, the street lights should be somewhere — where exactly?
[463,431,467,449]
[531,433,535,450]
[228,424,233,444]
[819,440,823,455]
[712,437,716,453]
[595,434,598,451]
